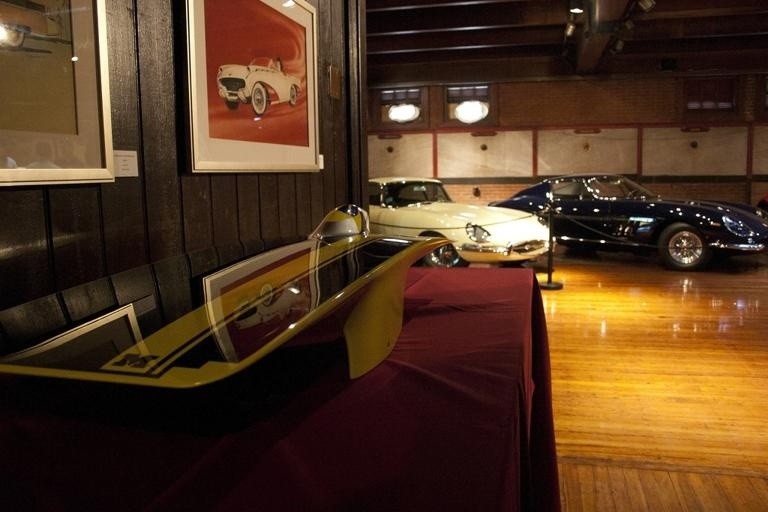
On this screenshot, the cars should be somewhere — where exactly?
[369,173,556,271]
[489,169,767,272]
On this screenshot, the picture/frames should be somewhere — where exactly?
[186,0,322,173]
[0,0,118,185]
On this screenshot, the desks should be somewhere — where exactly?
[0,265,561,512]
